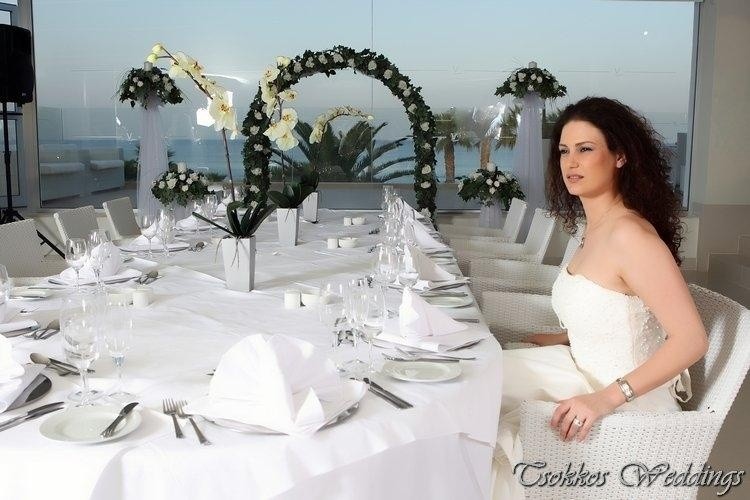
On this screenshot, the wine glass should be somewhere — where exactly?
[49,184,416,406]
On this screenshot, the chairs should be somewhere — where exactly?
[431,196,589,349]
[102,196,142,242]
[0,218,72,286]
[488,283,750,500]
[52,204,103,259]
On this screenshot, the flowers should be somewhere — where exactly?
[112,40,381,249]
[450,159,533,211]
[491,64,569,101]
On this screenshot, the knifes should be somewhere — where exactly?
[352,377,413,411]
[102,401,139,438]
[0,400,66,433]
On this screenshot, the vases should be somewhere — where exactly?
[218,236,259,294]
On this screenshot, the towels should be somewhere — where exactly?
[73,237,126,277]
[394,281,468,340]
[389,194,426,218]
[172,326,370,444]
[402,239,459,294]
[394,215,447,252]
[0,298,48,415]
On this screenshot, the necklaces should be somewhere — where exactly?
[578,197,623,248]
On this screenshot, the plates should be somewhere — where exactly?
[1,372,51,413]
[199,402,360,437]
[10,286,55,301]
[425,294,473,308]
[384,358,460,383]
[428,255,456,266]
[38,406,141,445]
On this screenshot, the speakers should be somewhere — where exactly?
[0,23,35,104]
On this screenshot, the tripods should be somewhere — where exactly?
[0,112,66,261]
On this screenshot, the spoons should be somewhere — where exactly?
[31,352,69,377]
[28,321,60,340]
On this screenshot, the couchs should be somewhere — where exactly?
[35,140,128,208]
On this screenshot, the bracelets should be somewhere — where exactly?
[616,377,635,402]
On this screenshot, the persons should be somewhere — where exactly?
[491,97,709,500]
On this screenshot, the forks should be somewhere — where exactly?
[383,353,474,365]
[161,398,184,439]
[174,401,211,446]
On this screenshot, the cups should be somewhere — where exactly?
[0,263,11,323]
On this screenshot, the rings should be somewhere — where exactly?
[573,418,583,427]
